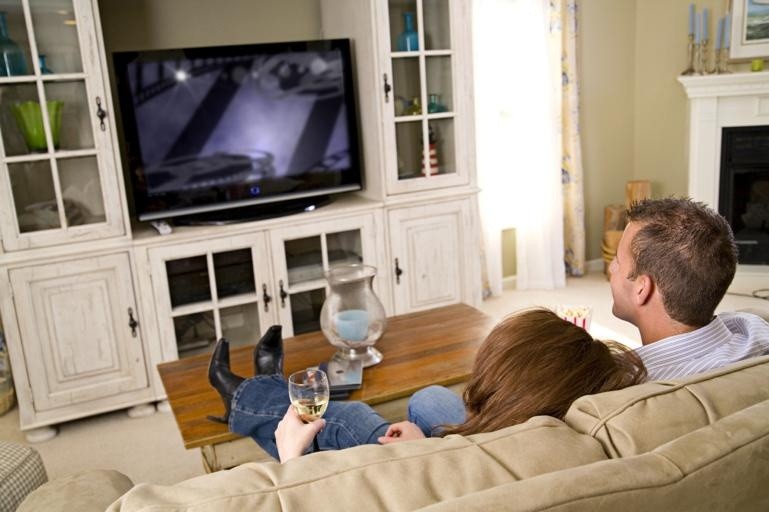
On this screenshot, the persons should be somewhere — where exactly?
[409,197,769,438]
[207,309,647,465]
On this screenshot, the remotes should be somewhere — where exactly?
[150,219,172,235]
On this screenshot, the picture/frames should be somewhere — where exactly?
[729,0,768,62]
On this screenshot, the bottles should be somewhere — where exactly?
[397,10,418,53]
[0,8,55,83]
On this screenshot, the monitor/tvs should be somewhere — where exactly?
[113,38,363,226]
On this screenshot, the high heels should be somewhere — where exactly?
[206,338,245,423]
[254,326,283,375]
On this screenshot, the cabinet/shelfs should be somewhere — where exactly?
[133,206,389,413]
[0,0,475,252]
[389,194,476,316]
[0,244,156,442]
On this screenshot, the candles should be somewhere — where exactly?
[688,5,730,54]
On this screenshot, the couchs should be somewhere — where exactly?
[16,354,767,512]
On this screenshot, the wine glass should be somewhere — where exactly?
[286,369,333,458]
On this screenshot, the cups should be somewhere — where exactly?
[329,307,370,343]
